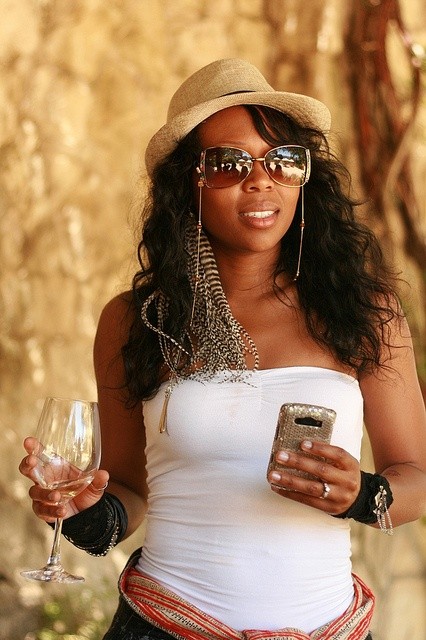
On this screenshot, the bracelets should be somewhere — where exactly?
[48,490,127,557]
[337,472,393,524]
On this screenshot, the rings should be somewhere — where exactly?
[319,483,332,501]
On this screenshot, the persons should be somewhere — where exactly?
[17,61,425,637]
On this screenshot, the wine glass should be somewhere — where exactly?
[20,396,101,584]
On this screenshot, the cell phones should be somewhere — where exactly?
[267,403,336,488]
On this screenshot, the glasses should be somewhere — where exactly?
[195,144,312,190]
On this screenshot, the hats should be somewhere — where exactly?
[144,58,332,183]
